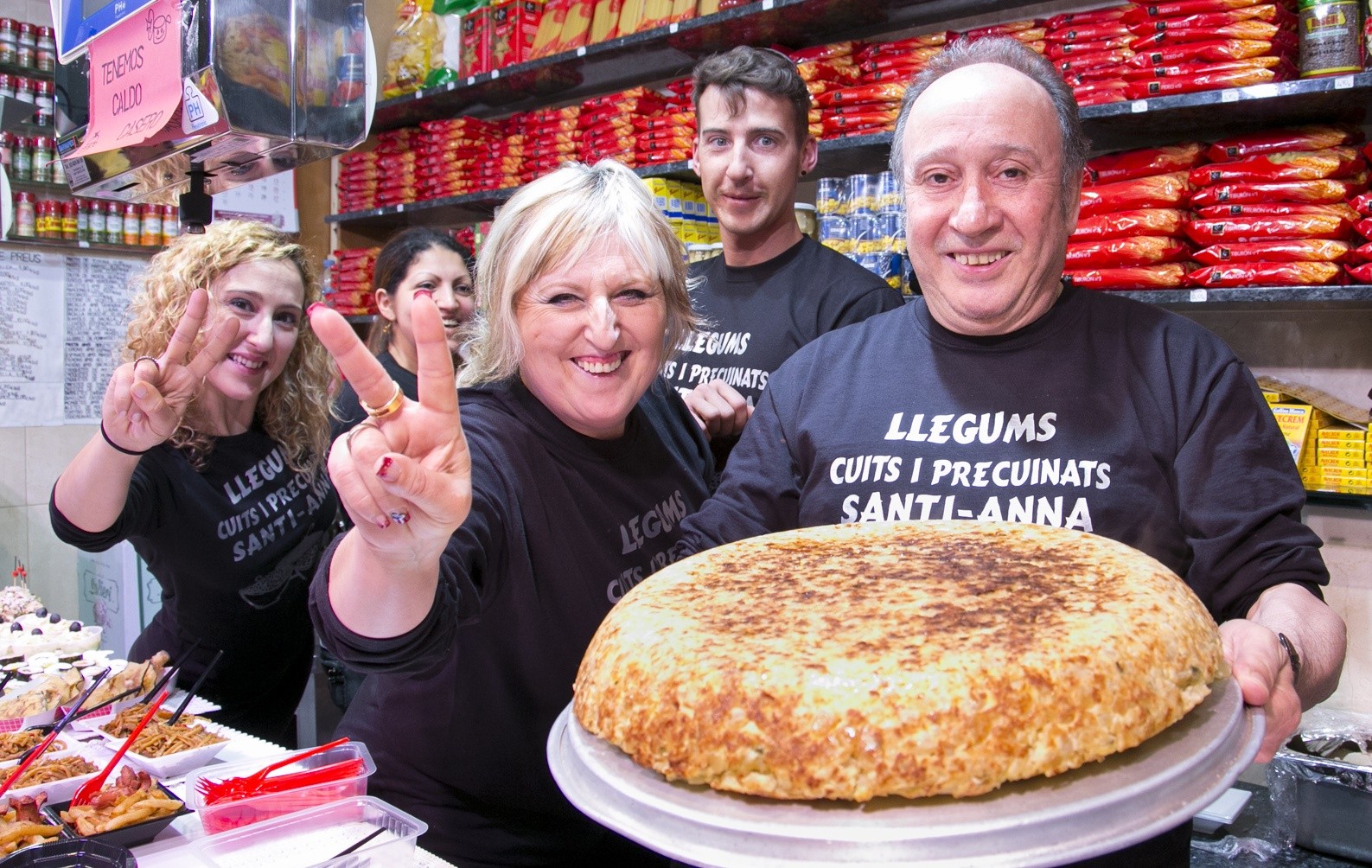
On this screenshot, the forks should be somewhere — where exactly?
[71,692,169,806]
[194,736,364,806]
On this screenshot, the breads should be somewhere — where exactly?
[574,518,1224,801]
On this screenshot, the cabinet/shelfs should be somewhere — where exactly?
[320,0,1372,505]
[0,63,171,255]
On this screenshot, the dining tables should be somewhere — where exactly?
[55,717,456,868]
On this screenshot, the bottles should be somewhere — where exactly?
[162,205,179,248]
[141,203,163,246]
[124,203,140,245]
[0,18,54,73]
[36,199,78,240]
[8,191,36,236]
[0,131,68,185]
[106,199,124,244]
[0,73,54,127]
[72,198,106,243]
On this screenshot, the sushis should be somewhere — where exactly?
[0,653,106,680]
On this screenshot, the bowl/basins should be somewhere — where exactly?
[80,705,232,778]
[0,838,139,868]
[0,731,116,811]
[43,780,186,849]
[0,612,58,661]
[189,795,428,868]
[185,741,377,836]
[41,620,103,654]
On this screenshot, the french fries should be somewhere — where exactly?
[60,776,183,836]
[0,810,63,859]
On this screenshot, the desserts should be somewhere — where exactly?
[0,585,103,657]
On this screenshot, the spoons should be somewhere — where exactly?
[206,787,349,833]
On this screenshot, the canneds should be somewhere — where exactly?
[794,172,923,296]
[334,3,365,105]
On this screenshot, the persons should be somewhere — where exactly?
[306,161,721,868]
[49,216,350,750]
[665,31,1351,868]
[656,44,906,482]
[323,230,476,457]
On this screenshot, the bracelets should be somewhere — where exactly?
[98,421,149,457]
[1277,633,1302,687]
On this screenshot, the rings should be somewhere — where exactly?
[133,356,160,372]
[360,381,404,419]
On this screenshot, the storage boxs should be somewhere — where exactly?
[488,0,543,70]
[458,6,488,79]
[1260,389,1372,496]
[642,178,721,264]
[185,739,376,840]
[186,795,429,868]
